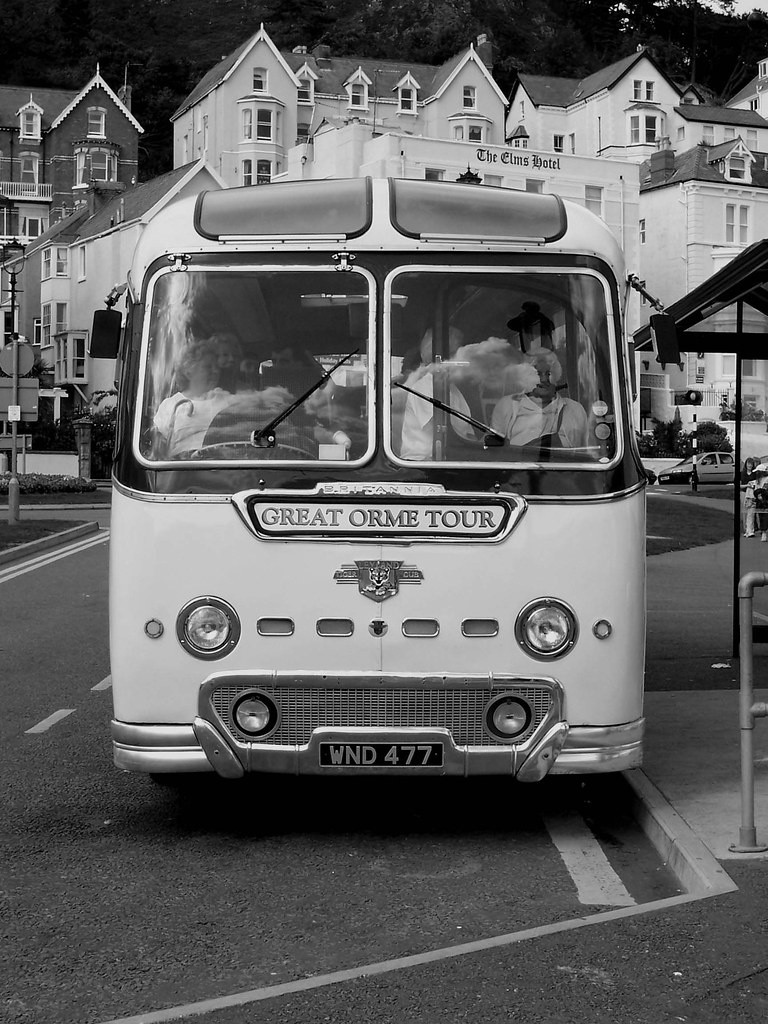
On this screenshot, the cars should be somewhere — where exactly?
[658,450,746,485]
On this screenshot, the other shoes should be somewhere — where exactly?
[746,533,756,538]
[743,533,747,537]
[761,534,767,542]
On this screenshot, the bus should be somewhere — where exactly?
[84,173,683,786]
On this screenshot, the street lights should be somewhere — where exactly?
[0,236,27,528]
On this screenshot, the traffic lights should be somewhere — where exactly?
[686,389,701,406]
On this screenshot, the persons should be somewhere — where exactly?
[148,330,329,464]
[485,347,590,454]
[739,456,768,542]
[400,322,479,460]
